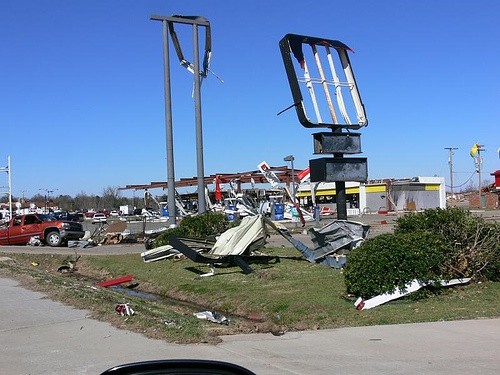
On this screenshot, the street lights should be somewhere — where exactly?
[284,154,295,202]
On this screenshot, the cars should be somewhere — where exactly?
[81,206,152,224]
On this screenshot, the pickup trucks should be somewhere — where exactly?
[0,211,85,247]
